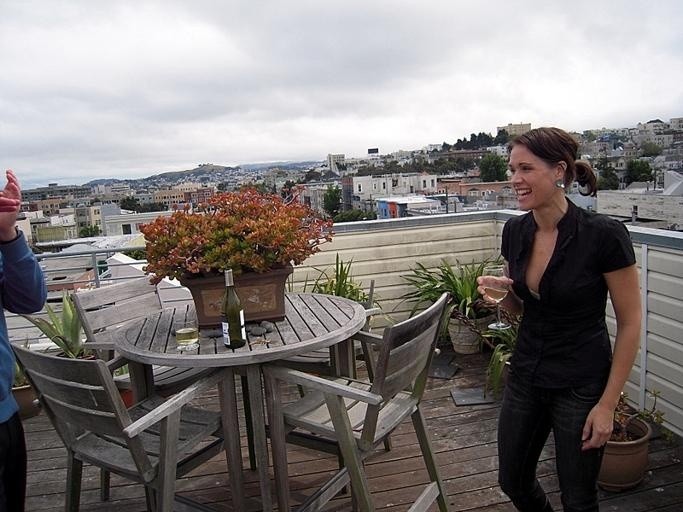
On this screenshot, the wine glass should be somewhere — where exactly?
[483,265,512,330]
[175,318,199,351]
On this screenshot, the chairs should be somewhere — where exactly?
[74,275,219,397]
[43,354,225,512]
[256,291,451,512]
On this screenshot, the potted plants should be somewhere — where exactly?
[599,389,677,494]
[396,257,498,354]
[41,289,96,360]
[141,188,337,329]
[12,338,44,421]
[114,365,134,409]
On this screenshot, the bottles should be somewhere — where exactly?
[220,268,247,350]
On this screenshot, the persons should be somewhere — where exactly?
[478,127,643,511]
[0,169,47,512]
